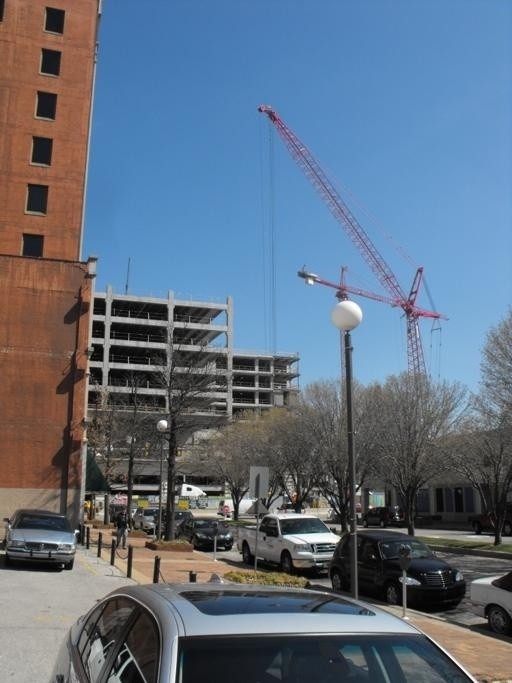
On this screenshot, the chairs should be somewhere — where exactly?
[289,651,364,683]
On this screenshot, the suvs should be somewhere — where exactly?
[132,506,159,534]
[327,529,467,610]
[362,506,404,527]
[471,501,512,536]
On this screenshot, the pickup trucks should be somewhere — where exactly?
[234,513,343,576]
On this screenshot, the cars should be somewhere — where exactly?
[49,583,481,682]
[3,509,82,570]
[176,517,233,550]
[469,568,512,634]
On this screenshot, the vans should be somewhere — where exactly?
[108,504,128,522]
[154,508,193,539]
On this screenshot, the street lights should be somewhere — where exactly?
[331,299,363,599]
[156,420,171,539]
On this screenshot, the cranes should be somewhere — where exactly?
[257,103,451,375]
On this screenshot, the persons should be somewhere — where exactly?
[114,507,133,550]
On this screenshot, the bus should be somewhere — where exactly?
[331,484,362,520]
